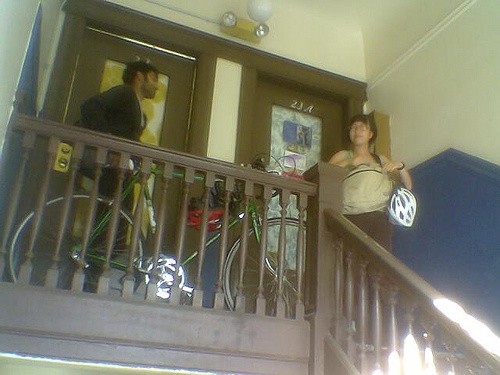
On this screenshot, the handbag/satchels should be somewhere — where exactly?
[67,121,126,180]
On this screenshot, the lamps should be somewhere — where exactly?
[247,1,273,21]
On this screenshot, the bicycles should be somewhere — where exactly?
[3,150,308,318]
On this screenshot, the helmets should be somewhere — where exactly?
[388,186,417,227]
[188,199,226,232]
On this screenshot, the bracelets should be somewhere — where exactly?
[397,161,405,171]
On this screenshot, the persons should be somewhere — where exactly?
[61,59,159,293]
[327,114,414,351]
